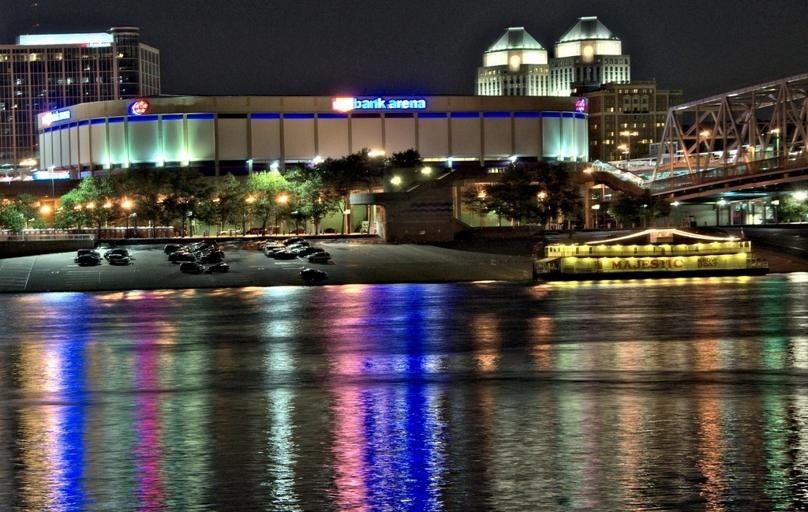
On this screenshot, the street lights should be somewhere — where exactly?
[51,165,56,197]
[772,198,779,222]
[592,204,601,229]
[74,194,287,239]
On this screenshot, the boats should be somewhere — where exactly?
[532,225,772,281]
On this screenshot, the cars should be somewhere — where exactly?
[324,227,336,233]
[299,267,329,281]
[73,247,132,266]
[163,237,231,275]
[289,228,304,233]
[256,237,333,264]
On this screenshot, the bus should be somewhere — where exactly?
[0,163,31,174]
